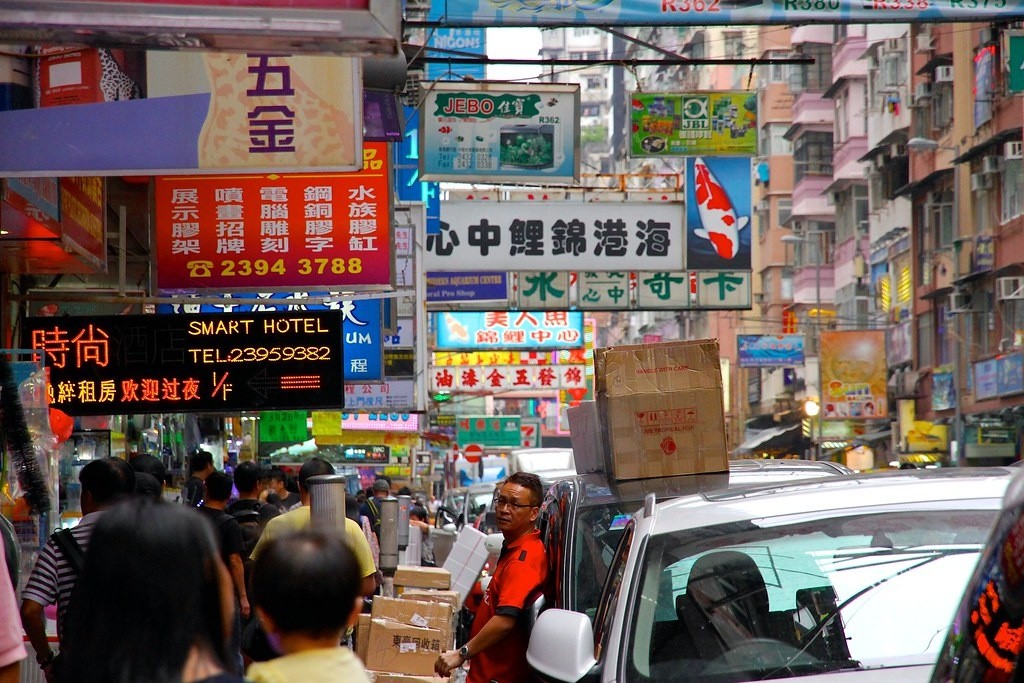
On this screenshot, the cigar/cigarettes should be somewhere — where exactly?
[433,672,438,677]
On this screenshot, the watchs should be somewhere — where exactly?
[459,643,470,661]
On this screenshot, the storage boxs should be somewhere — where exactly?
[592,340,730,479]
[351,565,458,683]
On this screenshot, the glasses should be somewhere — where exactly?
[493,499,540,511]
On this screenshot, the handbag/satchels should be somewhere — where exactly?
[242,615,282,661]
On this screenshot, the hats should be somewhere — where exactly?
[373,479,389,490]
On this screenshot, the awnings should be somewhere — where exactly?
[732,422,800,457]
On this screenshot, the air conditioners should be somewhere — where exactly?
[934,66,952,83]
[979,28,994,43]
[983,156,1005,175]
[906,82,932,109]
[995,276,1024,301]
[946,293,965,313]
[913,33,929,52]
[973,170,992,190]
[1005,141,1023,161]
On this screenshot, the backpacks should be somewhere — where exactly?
[51,528,88,683]
[366,499,381,540]
[231,502,268,561]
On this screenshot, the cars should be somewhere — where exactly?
[526,461,1024,683]
[927,477,1024,682]
[537,463,862,632]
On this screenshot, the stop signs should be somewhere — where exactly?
[463,444,484,463]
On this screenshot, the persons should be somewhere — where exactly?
[0,451,550,683]
[670,552,775,663]
